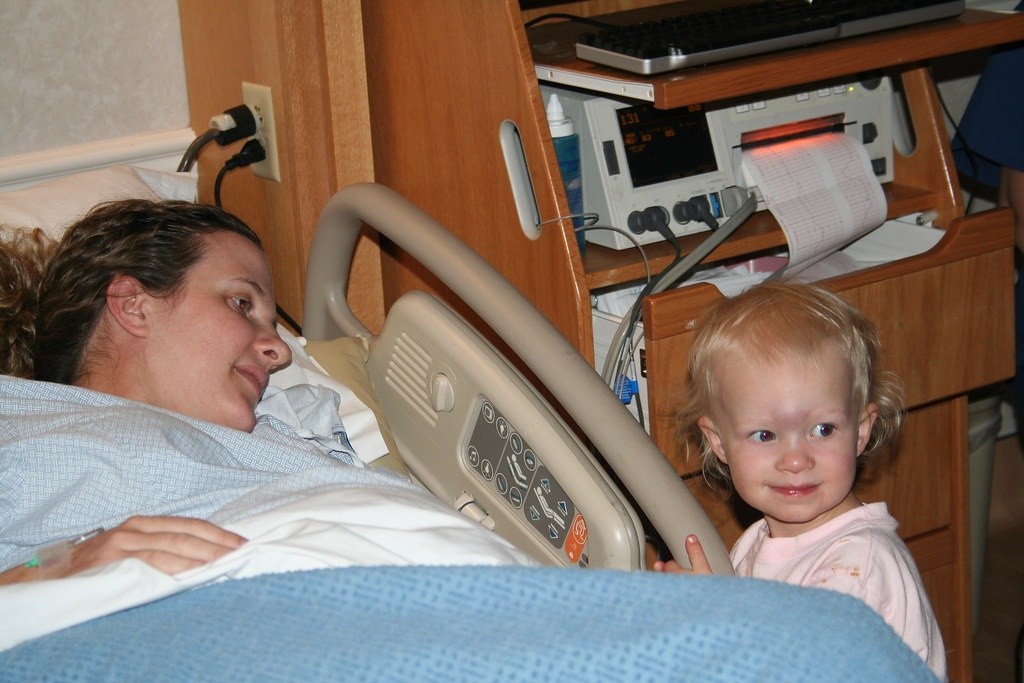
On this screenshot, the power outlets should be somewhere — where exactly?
[242,80,281,183]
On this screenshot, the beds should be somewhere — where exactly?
[0,159,941,683]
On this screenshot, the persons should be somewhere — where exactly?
[950,54,1024,422]
[653,281,952,683]
[0,197,410,586]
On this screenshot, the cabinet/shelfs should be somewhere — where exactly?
[358,0,1024,683]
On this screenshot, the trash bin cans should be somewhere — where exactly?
[967,394,1004,638]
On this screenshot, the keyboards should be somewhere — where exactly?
[574,0,965,76]
